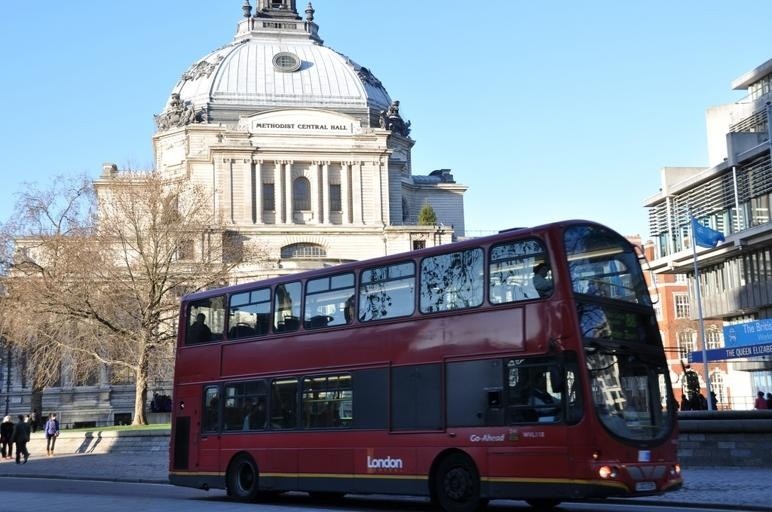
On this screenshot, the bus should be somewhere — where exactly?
[167,217,686,512]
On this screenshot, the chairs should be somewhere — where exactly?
[227,312,300,338]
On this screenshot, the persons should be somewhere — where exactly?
[532,263,554,298]
[25,413,31,422]
[32,408,39,432]
[192,312,211,339]
[207,392,341,430]
[529,373,561,416]
[585,276,606,298]
[10,414,31,465]
[44,412,60,458]
[665,389,771,411]
[1,414,15,460]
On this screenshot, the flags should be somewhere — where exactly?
[686,204,725,250]
